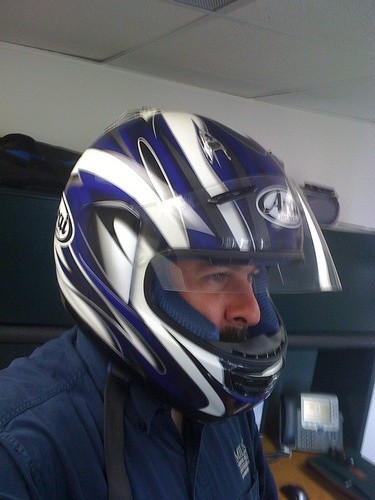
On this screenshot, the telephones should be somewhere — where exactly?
[275,392,344,455]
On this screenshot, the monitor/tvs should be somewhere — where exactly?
[353,367,375,471]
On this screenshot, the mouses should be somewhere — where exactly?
[280,484,308,500]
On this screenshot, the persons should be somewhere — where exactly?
[0,106,343,500]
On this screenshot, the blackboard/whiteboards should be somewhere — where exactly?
[1,188,375,354]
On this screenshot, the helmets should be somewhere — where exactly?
[53,107,343,420]
